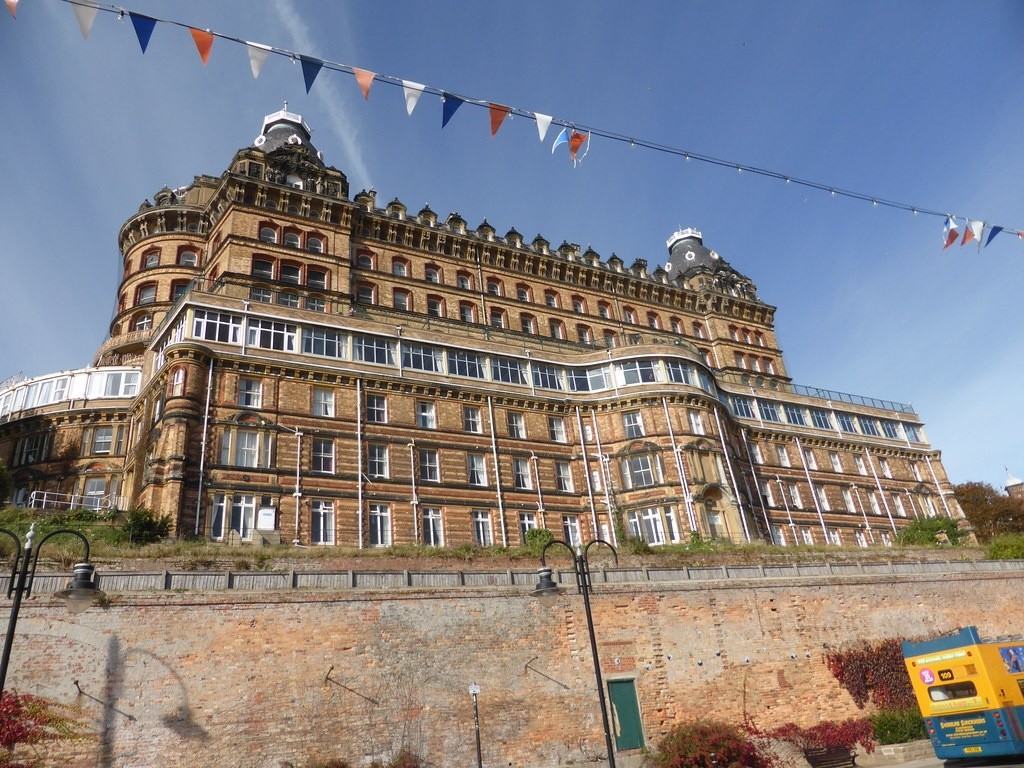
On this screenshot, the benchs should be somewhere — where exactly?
[804,744,859,768]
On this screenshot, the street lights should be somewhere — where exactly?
[529,540,619,768]
[0,523,107,699]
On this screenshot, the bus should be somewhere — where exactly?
[901,626,1024,759]
